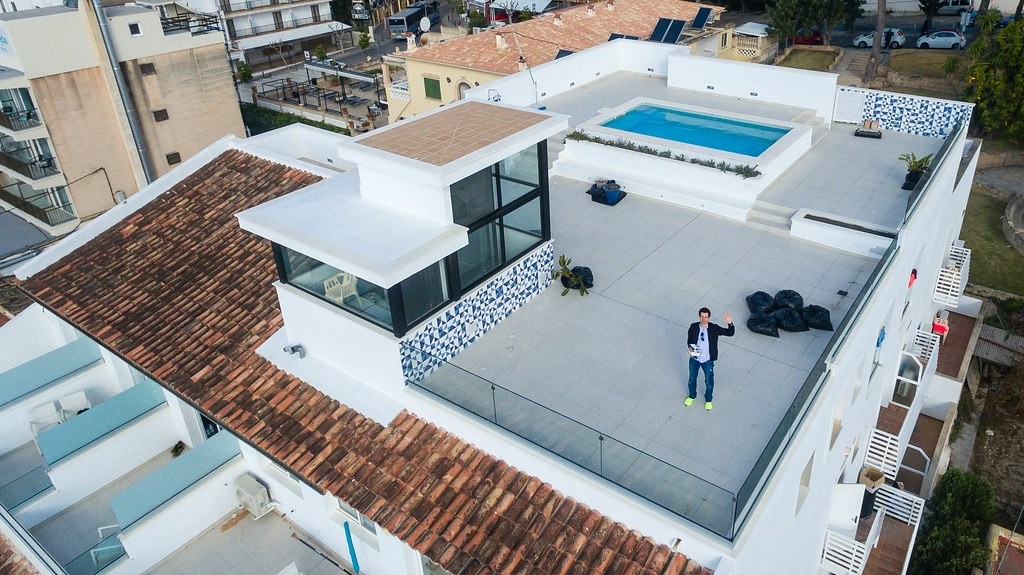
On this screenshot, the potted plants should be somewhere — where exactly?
[595,178,620,202]
[898,150,933,189]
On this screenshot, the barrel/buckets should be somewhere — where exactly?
[603,183,621,203]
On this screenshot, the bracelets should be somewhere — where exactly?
[728,322,733,326]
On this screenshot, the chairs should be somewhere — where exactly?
[300,87,369,107]
[350,82,385,95]
[934,315,949,345]
[28,402,62,456]
[18,110,35,127]
[324,272,364,309]
[30,154,44,171]
[59,391,91,421]
[39,156,54,174]
[89,525,123,566]
[1,106,13,118]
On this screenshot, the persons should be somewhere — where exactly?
[685,307,735,411]
[881,29,892,49]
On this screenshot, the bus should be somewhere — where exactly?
[388,0,441,42]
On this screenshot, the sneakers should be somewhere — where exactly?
[685,397,696,406]
[705,402,712,410]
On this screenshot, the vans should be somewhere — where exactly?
[936,0,974,17]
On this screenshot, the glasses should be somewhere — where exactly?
[701,332,705,341]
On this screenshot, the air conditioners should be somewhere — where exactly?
[897,371,915,397]
[237,474,269,518]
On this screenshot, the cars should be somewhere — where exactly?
[853,27,905,48]
[916,30,966,50]
[997,12,1024,28]
[787,26,829,46]
